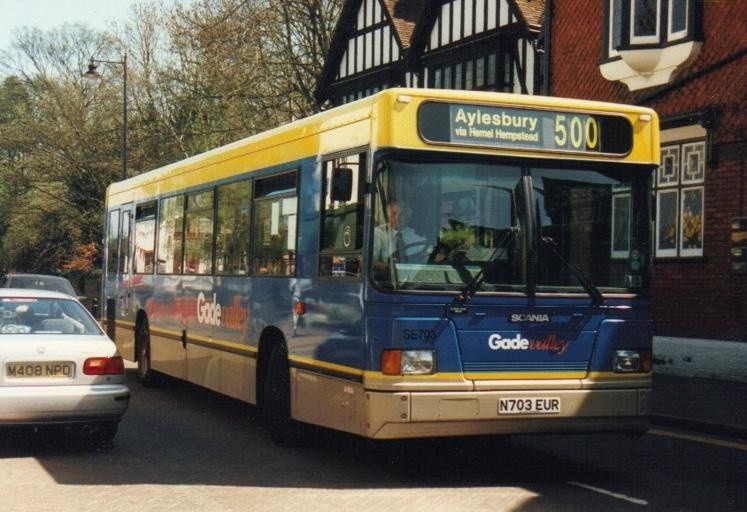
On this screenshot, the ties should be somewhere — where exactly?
[396,233,407,264]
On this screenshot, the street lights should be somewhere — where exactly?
[84,52,128,176]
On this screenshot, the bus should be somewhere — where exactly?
[101,86,663,450]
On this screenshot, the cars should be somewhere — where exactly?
[0,288,131,444]
[0,274,80,303]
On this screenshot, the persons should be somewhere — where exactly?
[372,200,428,279]
